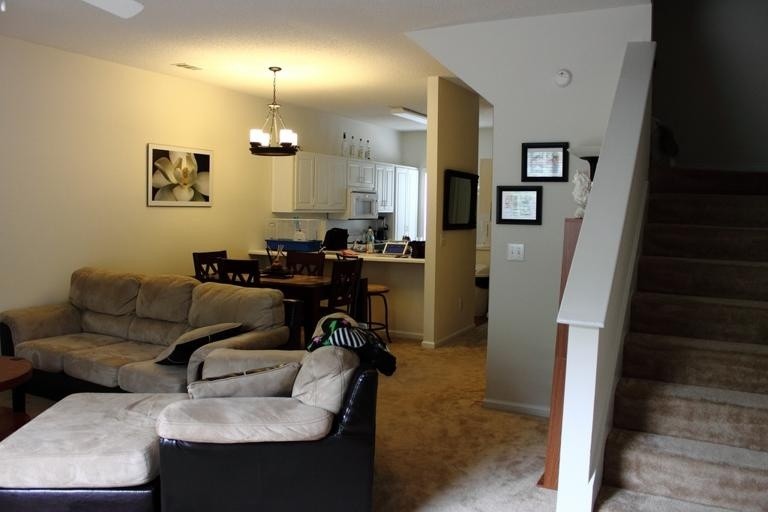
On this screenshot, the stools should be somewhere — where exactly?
[367,284,391,342]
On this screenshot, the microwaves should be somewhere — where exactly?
[328,192,379,221]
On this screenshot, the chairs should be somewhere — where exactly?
[192,249,368,314]
[157,347,378,512]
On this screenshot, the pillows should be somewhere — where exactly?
[187,363,299,399]
[156,323,244,364]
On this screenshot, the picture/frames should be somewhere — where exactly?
[521,142,569,182]
[497,185,543,224]
[148,143,213,207]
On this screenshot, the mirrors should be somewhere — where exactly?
[443,170,479,231]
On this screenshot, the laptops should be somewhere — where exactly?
[376,242,408,258]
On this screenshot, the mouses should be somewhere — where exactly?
[402,255,408,258]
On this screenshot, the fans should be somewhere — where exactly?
[85,0,143,20]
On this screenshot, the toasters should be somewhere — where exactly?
[326,228,347,250]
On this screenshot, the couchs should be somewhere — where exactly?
[0,266,289,400]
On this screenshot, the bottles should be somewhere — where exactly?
[358,139,365,160]
[350,136,356,158]
[367,227,374,252]
[341,132,348,158]
[366,140,372,161]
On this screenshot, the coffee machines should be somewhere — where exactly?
[374,216,388,242]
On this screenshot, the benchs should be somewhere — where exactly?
[0,393,188,511]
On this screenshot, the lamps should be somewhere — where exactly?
[249,67,299,157]
[391,108,427,126]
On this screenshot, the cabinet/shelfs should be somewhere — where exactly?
[375,162,395,213]
[272,153,347,214]
[385,164,419,241]
[347,158,376,191]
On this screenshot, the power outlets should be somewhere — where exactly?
[507,243,525,262]
